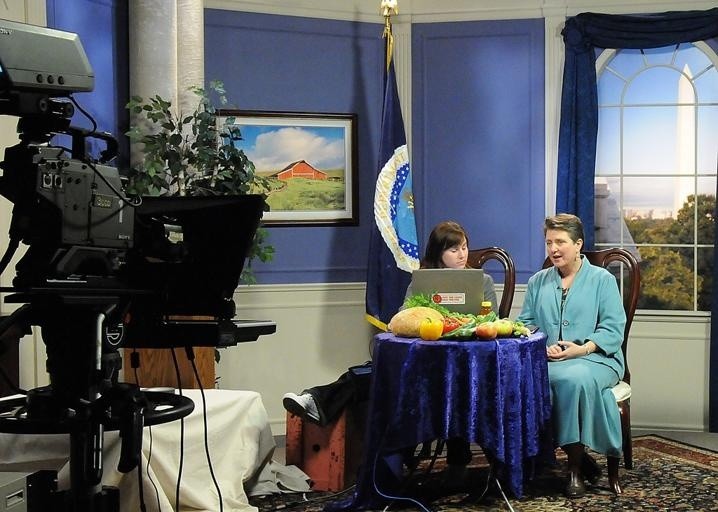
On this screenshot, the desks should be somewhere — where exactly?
[373,331,553,489]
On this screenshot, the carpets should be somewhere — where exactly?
[249,434,718,512]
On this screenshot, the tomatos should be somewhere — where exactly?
[442,319,458,333]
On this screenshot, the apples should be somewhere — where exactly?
[476,319,512,339]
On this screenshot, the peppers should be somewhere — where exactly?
[419,317,444,341]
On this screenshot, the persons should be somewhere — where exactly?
[398,221,501,486]
[517,213,628,499]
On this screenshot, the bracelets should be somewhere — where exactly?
[583,342,591,356]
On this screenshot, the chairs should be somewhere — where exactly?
[541,247,640,494]
[418,247,515,461]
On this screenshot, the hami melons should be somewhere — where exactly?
[388,307,446,338]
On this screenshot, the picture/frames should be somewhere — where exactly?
[206,109,359,228]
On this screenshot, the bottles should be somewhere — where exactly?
[481,302,492,315]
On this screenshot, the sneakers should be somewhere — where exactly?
[282,392,320,425]
[586,457,602,485]
[433,467,461,485]
[565,471,585,498]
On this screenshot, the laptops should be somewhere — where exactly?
[412,270,483,315]
